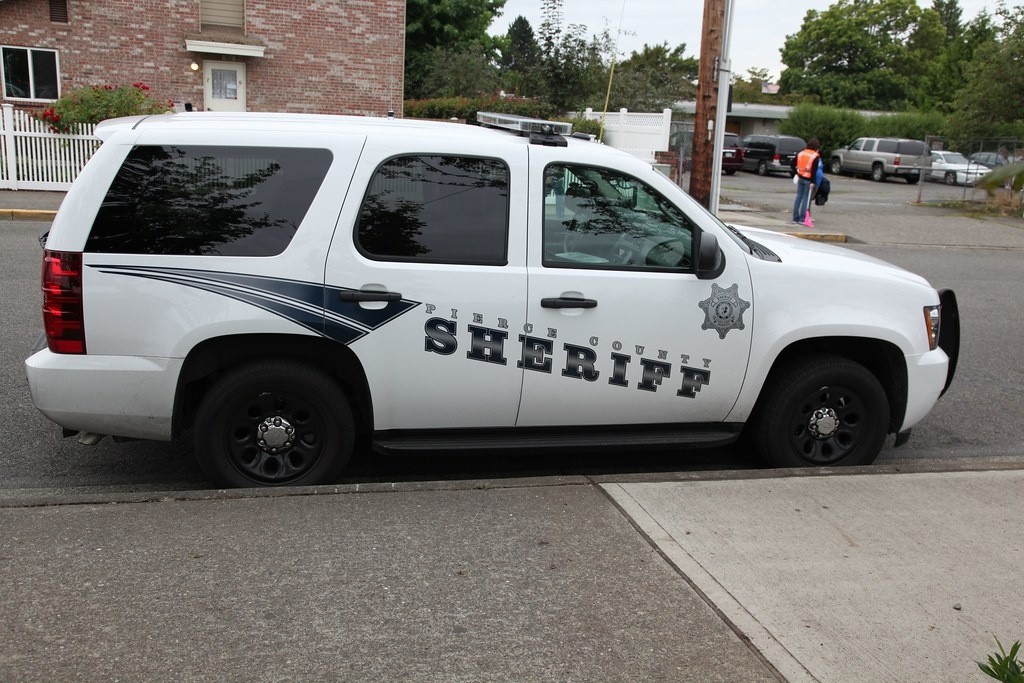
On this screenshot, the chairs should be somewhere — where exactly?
[439,170,506,260]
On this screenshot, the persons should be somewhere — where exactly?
[791,138,823,224]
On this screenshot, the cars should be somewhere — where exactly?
[20,111,963,490]
[928,150,993,187]
[969,152,1024,190]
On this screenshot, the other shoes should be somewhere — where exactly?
[799,221,805,225]
[793,220,799,224]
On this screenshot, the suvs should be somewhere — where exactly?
[741,133,808,177]
[828,138,933,183]
[671,129,744,175]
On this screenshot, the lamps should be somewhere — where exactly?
[191,60,199,71]
